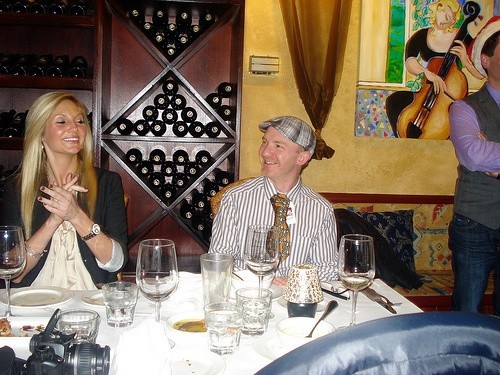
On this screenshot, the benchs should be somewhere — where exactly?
[211,177,500,316]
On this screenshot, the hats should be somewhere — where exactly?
[258,115,317,157]
[467,15,500,79]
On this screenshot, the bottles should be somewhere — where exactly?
[0,0,237,243]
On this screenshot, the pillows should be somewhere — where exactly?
[353,210,416,274]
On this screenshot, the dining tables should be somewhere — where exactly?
[0,278,424,375]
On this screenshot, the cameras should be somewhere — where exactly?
[0,308,111,375]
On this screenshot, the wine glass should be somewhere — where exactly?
[0,225,27,318]
[136,239,179,349]
[244,224,277,294]
[337,234,376,331]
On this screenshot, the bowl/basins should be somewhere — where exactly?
[276,317,335,346]
[233,270,272,293]
[167,311,209,341]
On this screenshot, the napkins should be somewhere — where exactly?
[108,315,173,375]
[165,270,236,310]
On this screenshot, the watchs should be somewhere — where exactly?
[80,224,101,242]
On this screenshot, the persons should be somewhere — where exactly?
[205,116,340,286]
[0,91,128,288]
[446,15,500,316]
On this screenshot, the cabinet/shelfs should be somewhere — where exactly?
[0,0,246,282]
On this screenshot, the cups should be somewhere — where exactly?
[200,254,235,303]
[284,265,323,304]
[59,309,100,345]
[235,288,272,336]
[287,302,318,318]
[102,282,139,327]
[204,302,244,357]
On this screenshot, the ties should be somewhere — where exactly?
[267,193,290,264]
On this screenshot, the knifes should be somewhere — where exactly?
[362,288,397,315]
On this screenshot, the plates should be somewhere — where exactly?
[255,330,291,359]
[0,286,73,308]
[82,291,107,307]
[0,316,51,340]
[263,283,286,302]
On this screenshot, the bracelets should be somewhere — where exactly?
[25,240,43,259]
